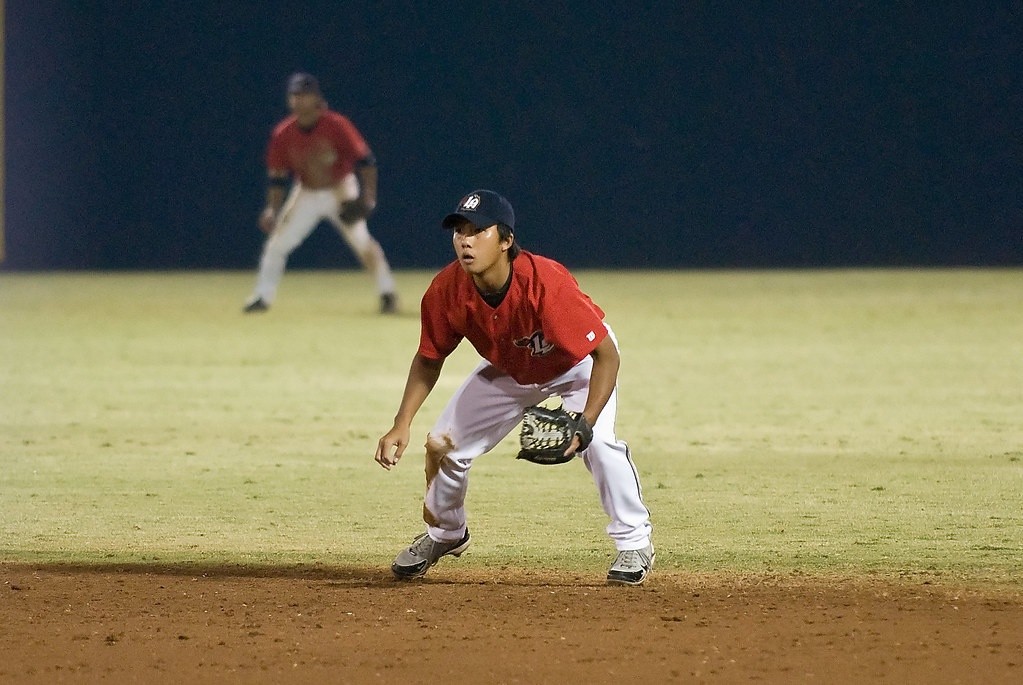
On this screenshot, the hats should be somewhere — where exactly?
[287,73,320,94]
[442,190,515,233]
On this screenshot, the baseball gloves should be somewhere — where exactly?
[515,406,594,465]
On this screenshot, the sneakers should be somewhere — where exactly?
[391,527,471,578]
[381,291,396,313]
[606,541,655,585]
[244,297,268,312]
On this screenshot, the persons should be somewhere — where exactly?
[374,189,656,585]
[238,73,398,316]
